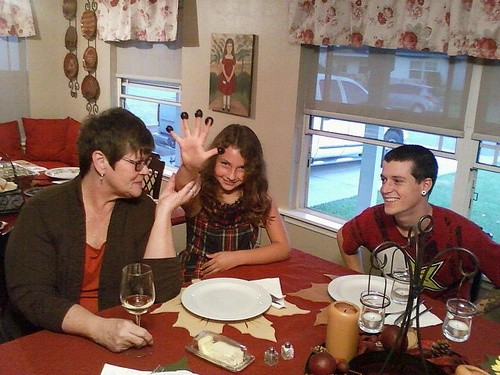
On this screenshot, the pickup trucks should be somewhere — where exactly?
[154,74,406,167]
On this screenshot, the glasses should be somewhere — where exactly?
[122,154,152,171]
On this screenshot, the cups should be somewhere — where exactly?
[359,272,479,342]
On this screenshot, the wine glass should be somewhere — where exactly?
[120,263,155,327]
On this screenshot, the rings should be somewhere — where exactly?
[190,189,195,196]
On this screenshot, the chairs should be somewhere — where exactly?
[141,157,164,201]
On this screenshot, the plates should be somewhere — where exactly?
[181,277,272,322]
[327,273,418,314]
[45,167,80,180]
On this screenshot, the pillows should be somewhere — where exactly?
[64,118,85,165]
[21,117,66,161]
[0,120,24,160]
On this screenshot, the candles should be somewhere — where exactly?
[326,302,360,361]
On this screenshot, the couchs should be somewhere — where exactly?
[0,117,81,174]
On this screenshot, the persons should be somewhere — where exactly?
[0,107,202,352]
[166,109,292,285]
[336,144,500,316]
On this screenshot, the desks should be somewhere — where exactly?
[0,242,500,375]
[0,160,186,243]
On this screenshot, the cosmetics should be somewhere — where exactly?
[281,342,294,360]
[264,347,278,366]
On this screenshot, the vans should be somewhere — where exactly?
[377,79,442,118]
